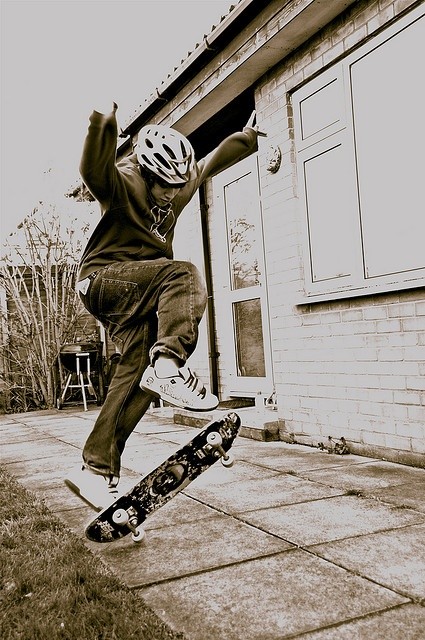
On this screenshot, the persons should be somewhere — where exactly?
[64,104,259,515]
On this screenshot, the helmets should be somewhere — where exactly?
[136,124,194,187]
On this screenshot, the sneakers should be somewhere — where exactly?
[66,467,122,513]
[139,364,219,411]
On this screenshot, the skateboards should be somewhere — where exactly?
[84,411,241,544]
